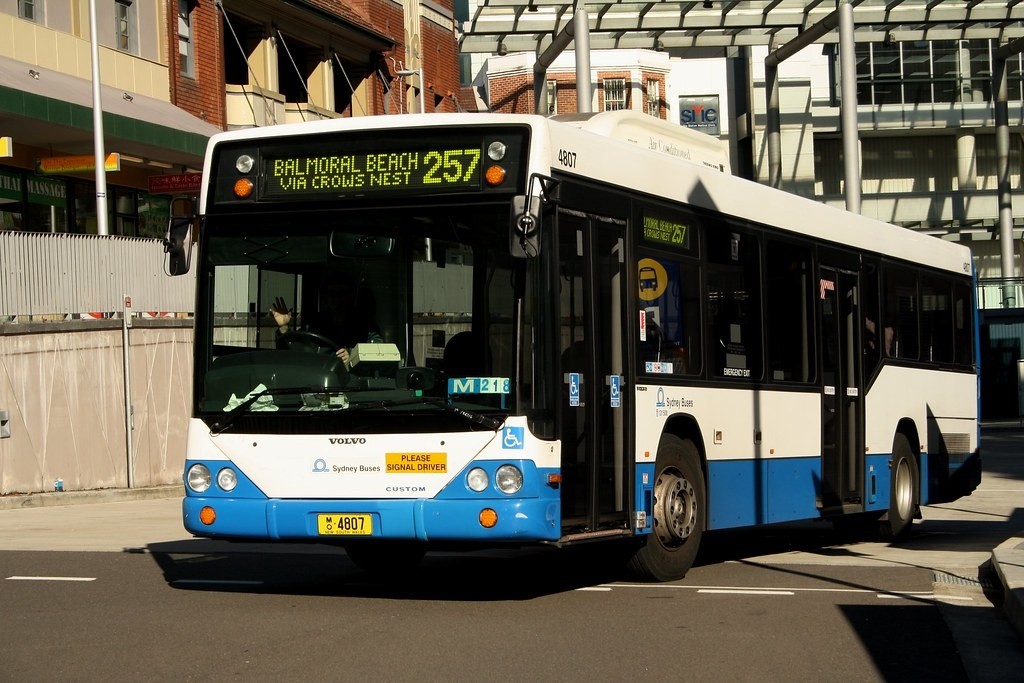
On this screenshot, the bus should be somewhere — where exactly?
[163,103,983,585]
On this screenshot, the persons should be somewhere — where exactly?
[270,265,383,368]
